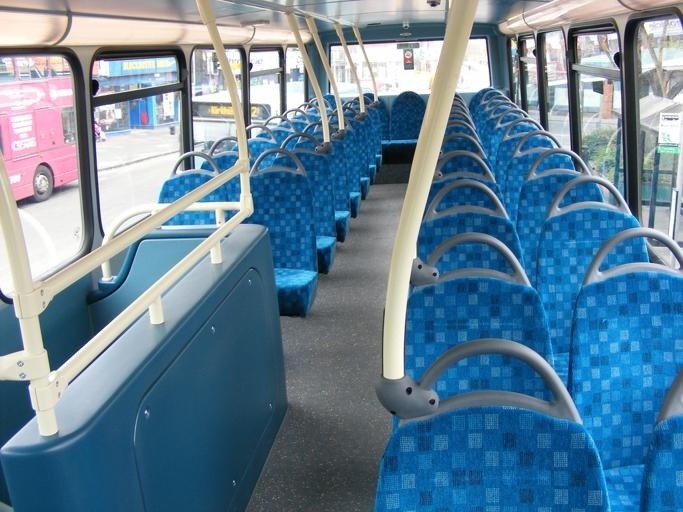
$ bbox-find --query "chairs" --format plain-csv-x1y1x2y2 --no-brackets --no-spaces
377,90,682,511
157,92,383,318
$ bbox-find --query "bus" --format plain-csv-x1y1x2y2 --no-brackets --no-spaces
169,81,305,168
0,77,79,203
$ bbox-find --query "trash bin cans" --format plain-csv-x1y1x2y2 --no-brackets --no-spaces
600,78,615,120
